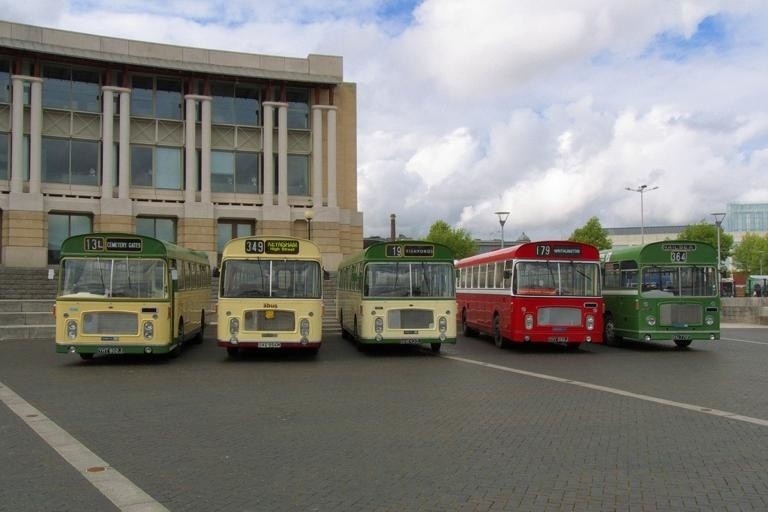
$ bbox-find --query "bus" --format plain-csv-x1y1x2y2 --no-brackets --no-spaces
452,239,606,352
596,239,720,347
47,232,212,358
212,234,330,356
334,238,460,352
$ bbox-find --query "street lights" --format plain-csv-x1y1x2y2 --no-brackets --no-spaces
494,211,512,247
304,210,314,239
710,212,726,276
625,184,659,242
